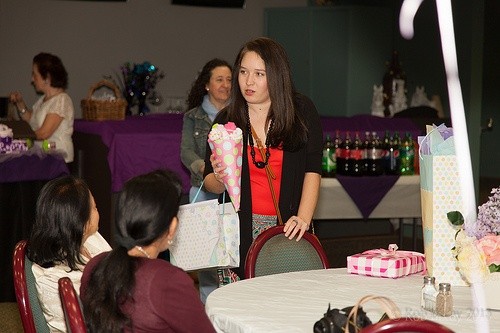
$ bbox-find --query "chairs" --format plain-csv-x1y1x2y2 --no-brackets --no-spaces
13,241,48,333
59,277,87,333
245,226,328,282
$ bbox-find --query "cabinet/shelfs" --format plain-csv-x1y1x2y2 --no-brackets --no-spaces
266,4,394,118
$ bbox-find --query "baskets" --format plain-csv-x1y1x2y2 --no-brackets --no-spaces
80,80,127,121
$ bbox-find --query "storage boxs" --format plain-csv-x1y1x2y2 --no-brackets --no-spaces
347,248,425,278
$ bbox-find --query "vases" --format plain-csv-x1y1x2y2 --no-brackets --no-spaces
483,272,500,311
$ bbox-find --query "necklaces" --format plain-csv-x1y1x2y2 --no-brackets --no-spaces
244,101,277,168
135,246,151,259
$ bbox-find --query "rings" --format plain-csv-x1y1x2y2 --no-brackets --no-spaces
293,219,298,226
213,170,217,175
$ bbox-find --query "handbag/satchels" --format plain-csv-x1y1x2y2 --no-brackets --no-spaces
313,303,373,333
418,123,472,287
168,173,241,272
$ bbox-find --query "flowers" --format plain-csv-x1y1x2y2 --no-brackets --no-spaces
465,188,500,273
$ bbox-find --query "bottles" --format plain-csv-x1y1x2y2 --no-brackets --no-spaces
436,283,452,316
321,131,416,178
421,276,437,311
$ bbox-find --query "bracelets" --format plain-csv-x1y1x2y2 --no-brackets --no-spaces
18,106,28,114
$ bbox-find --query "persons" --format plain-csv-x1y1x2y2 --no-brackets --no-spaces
9,52,75,172
80,172,217,333
179,37,323,281
23,171,100,333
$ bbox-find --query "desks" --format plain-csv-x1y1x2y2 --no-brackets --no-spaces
204,268,500,333
75,115,428,250
0,150,69,239
